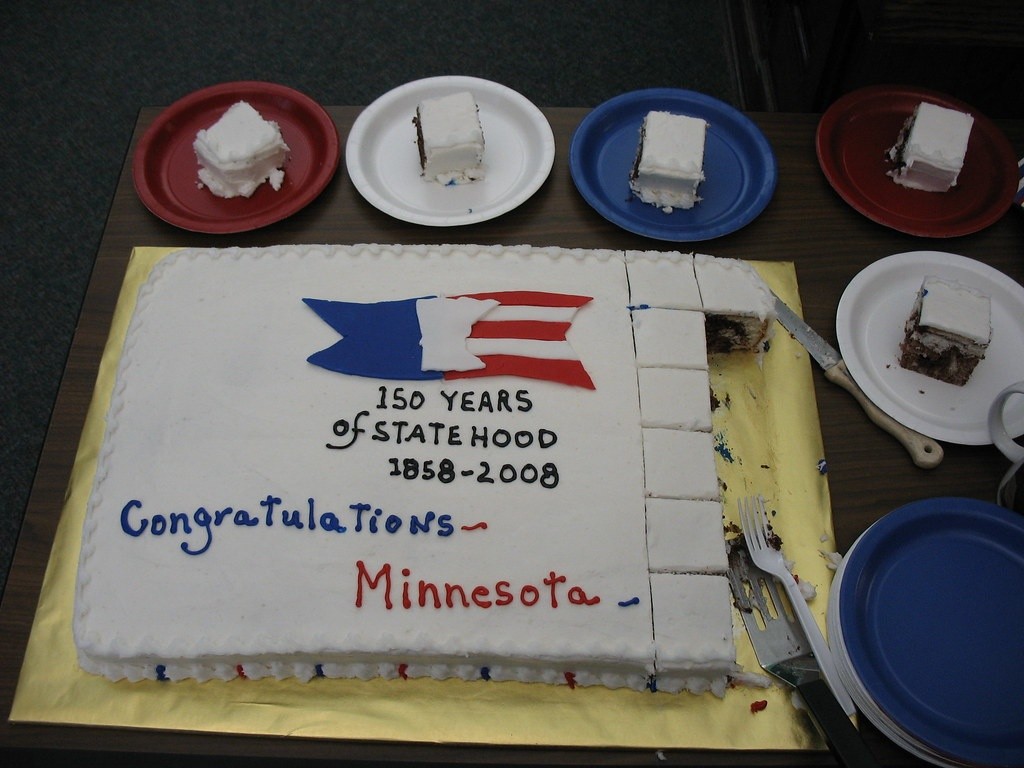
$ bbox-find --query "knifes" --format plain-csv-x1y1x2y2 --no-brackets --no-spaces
771,288,944,469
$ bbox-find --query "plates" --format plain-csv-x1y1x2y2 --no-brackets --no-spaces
836,251,1024,445
346,75,554,227
570,88,777,241
815,84,1019,237
826,496,1024,768
132,81,339,233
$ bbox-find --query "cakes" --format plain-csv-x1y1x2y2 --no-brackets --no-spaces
888,100,975,193
899,275,994,387
630,109,710,214
71,243,778,696
193,97,293,201
413,90,490,184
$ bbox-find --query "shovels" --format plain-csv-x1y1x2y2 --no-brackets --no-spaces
726,537,885,768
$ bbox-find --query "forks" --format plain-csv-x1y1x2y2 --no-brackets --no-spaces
736,496,856,717
727,564,880,768
